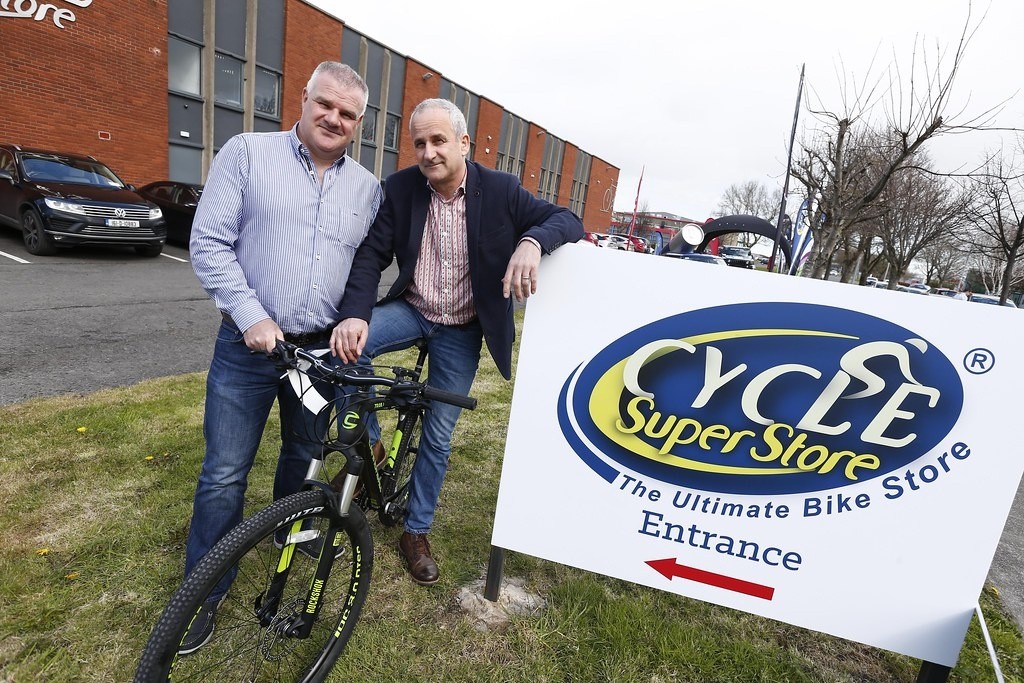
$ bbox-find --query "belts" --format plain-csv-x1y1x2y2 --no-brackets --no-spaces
220,310,333,347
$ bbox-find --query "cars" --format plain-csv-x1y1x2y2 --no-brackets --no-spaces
586,232,651,250
865,276,1018,308
0,144,168,257
721,246,756,267
131,181,205,241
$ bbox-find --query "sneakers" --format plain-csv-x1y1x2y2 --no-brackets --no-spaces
177,589,232,654
273,527,345,561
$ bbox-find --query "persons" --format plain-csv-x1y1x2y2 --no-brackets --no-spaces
954,288,972,302
328,98,585,587
173,60,387,656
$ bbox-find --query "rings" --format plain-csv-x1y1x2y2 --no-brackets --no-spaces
522,277,530,279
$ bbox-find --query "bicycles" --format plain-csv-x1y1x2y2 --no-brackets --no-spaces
134,338,476,683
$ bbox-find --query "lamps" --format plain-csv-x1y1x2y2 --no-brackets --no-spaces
422,72,433,81
535,131,544,136
606,166,611,171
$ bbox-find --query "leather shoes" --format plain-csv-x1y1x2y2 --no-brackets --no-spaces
398,530,440,585
328,438,386,500
